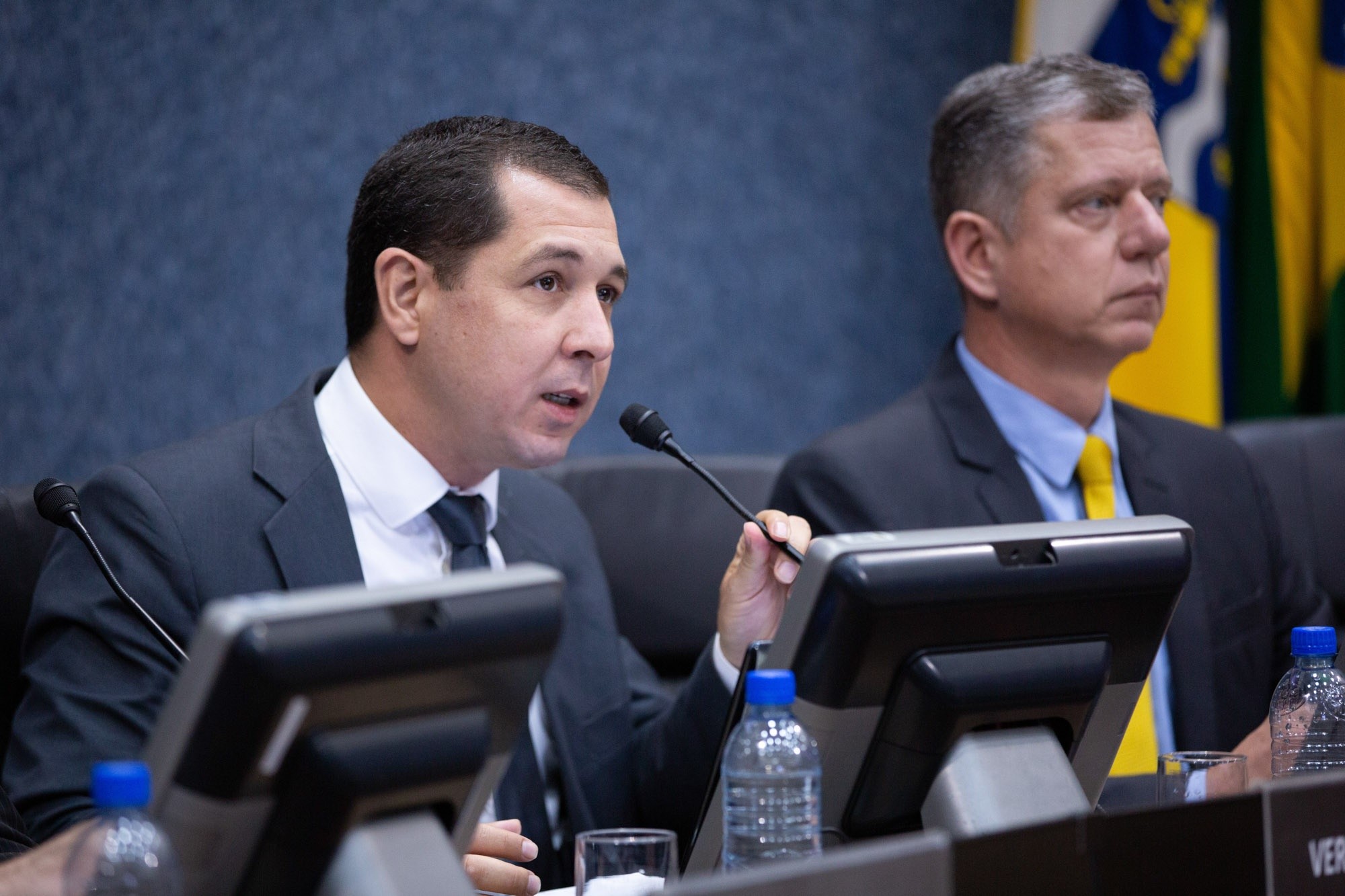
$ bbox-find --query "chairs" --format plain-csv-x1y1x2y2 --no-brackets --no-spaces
559,458,791,701
1227,412,1345,690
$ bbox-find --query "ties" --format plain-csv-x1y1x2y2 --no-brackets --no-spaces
425,489,571,892
1076,435,1159,777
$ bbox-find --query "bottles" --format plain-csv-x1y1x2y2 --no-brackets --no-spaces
719,670,822,874
62,760,186,896
1268,625,1345,781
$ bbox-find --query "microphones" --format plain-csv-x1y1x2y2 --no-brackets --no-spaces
620,403,808,565
31,478,189,665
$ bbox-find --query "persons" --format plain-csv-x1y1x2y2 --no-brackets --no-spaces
766,49,1345,813
0,114,810,896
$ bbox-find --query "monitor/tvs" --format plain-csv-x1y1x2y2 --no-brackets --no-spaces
681,515,1193,880
135,560,566,896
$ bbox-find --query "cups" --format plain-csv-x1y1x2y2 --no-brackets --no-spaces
1157,750,1248,807
573,829,680,895
